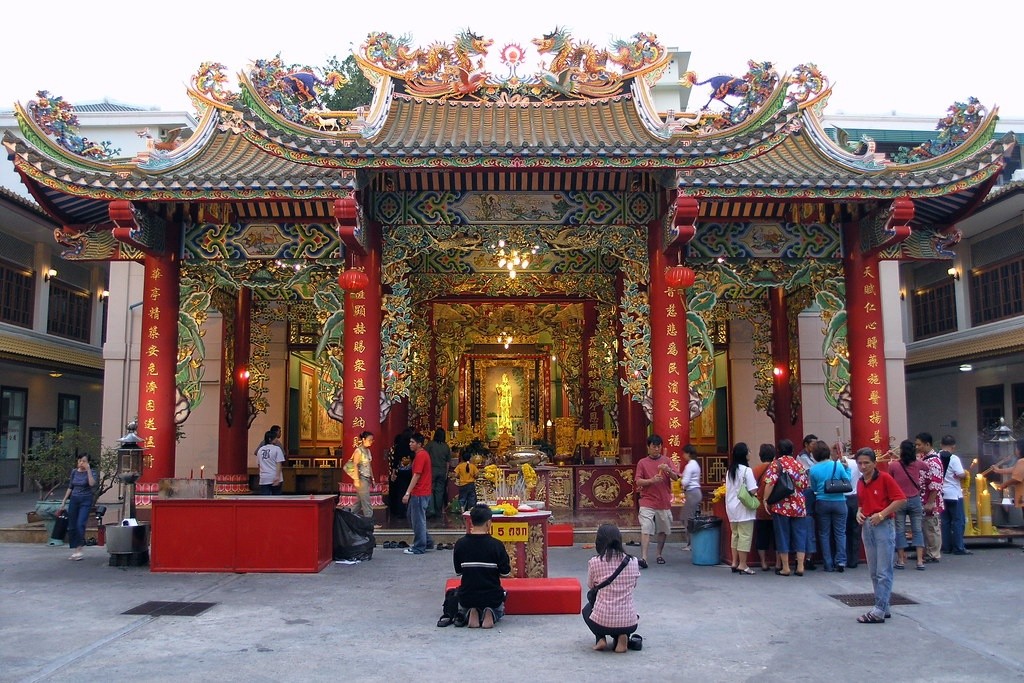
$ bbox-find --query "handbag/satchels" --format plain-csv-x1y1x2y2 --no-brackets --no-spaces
767,459,795,504
51,512,68,540
825,460,852,493
738,467,760,511
587,587,598,606
343,448,362,479
442,585,468,617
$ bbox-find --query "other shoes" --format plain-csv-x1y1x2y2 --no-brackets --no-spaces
638,557,647,568
382,540,409,549
445,543,453,550
922,554,939,564
955,549,973,556
69,554,85,560
656,557,666,564
731,557,857,577
908,552,917,559
437,542,444,550
404,549,414,554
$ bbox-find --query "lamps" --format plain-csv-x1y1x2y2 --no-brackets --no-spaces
44,270,57,282
947,268,959,281
99,291,109,302
490,231,540,278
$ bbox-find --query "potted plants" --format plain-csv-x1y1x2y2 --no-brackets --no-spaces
21,424,119,547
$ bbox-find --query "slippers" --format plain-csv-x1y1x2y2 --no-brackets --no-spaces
916,563,926,569
893,561,906,570
625,540,640,546
455,610,467,626
436,612,454,627
856,613,885,624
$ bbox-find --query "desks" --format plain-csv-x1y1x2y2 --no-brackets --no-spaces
446,465,635,579
282,467,344,494
150,495,339,573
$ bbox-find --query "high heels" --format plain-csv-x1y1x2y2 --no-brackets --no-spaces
630,633,642,650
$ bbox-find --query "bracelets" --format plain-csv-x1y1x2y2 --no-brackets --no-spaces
666,468,672,477
878,513,885,521
762,498,768,502
371,478,375,481
354,478,359,482
405,492,410,497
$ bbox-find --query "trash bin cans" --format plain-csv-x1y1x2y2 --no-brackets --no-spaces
689,515,721,566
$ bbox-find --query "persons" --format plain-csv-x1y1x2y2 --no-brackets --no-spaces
61,453,98,560
724,434,968,576
254,425,286,495
350,431,382,529
634,434,702,569
454,504,511,628
388,427,451,518
582,523,641,653
402,433,435,554
854,448,908,623
991,440,1024,553
495,373,512,436
454,451,479,513
